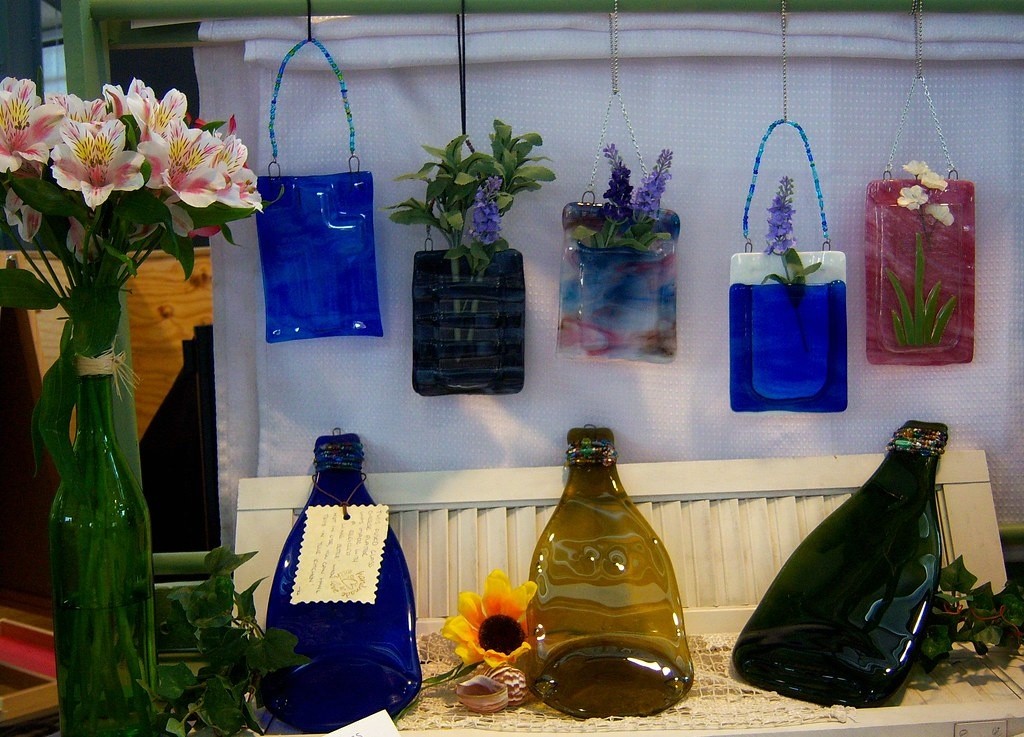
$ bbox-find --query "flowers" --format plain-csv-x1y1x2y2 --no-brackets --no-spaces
896,159,954,250
760,175,822,353
389,570,539,729
571,142,674,256
0,77,263,737
386,117,556,364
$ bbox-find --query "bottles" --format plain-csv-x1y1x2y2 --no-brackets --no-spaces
525,428,693,717
732,421,949,709
258,432,421,732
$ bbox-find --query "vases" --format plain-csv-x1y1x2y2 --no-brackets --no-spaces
48,351,154,736
